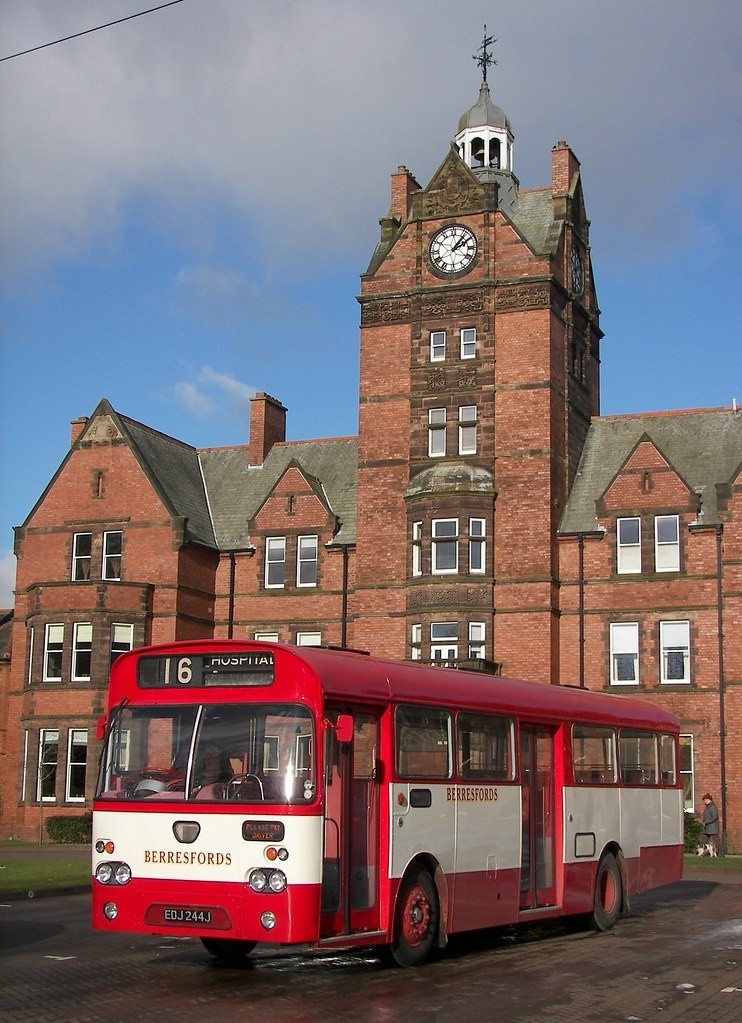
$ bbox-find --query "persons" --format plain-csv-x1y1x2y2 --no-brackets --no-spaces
694,794,722,853
168,719,233,790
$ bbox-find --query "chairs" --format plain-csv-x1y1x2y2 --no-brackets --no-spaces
462,763,673,825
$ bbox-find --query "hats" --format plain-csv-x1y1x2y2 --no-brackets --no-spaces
702,793,712,800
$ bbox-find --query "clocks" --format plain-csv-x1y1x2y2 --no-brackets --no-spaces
430,225,478,274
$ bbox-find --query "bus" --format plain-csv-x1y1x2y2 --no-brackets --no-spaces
86,637,689,964
